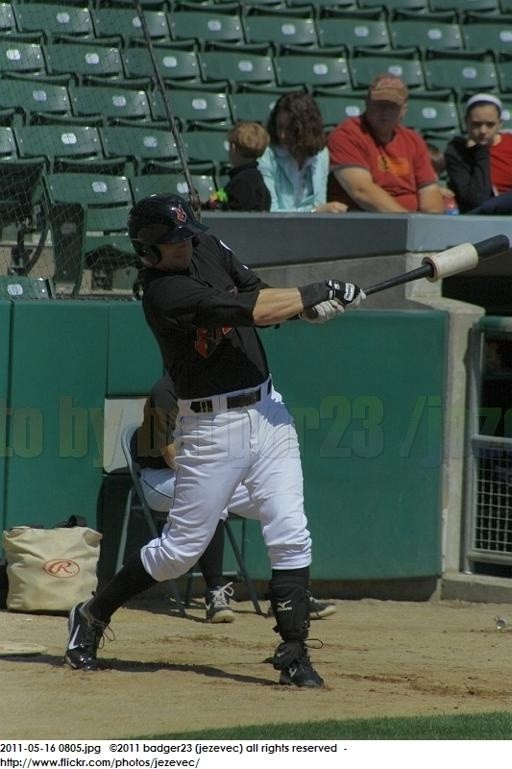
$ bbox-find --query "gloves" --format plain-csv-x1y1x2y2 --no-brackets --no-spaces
297,277,366,310
288,300,345,325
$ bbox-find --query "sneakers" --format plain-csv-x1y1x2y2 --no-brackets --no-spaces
266,598,337,620
278,656,324,688
203,578,238,623
65,598,118,671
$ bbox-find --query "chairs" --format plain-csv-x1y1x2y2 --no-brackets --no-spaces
113,422,267,619
1,1,511,286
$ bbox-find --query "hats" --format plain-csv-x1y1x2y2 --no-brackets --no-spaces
367,75,409,108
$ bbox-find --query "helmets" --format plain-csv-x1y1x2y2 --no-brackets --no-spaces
126,193,209,261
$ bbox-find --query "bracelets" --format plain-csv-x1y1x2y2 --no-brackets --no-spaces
297,280,331,309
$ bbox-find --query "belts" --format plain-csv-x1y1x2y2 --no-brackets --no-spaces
189,379,272,414
142,458,170,470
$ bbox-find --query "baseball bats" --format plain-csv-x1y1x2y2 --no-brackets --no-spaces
307,233,509,320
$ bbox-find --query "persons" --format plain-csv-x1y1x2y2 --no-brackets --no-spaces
257,92,349,214
446,94,512,216
135,372,336,623
65,192,367,690
426,143,460,216
207,121,272,212
326,77,456,215
